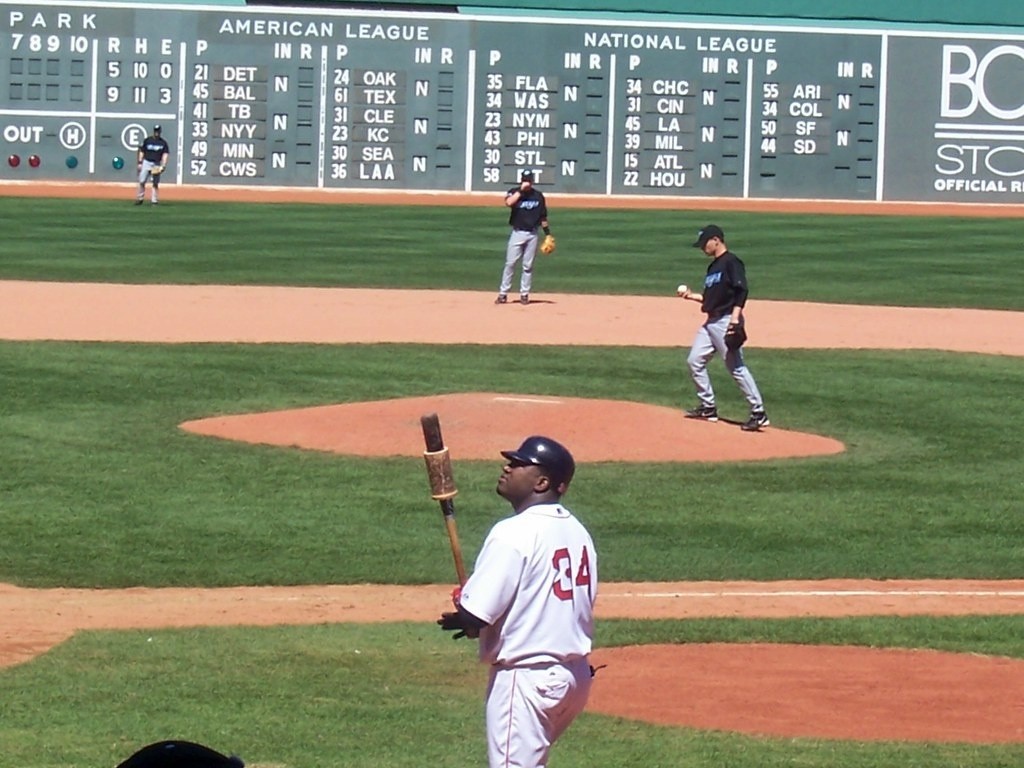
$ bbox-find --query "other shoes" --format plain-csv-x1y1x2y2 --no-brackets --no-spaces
152,202,158,206
134,200,143,205
521,294,529,305
495,294,507,304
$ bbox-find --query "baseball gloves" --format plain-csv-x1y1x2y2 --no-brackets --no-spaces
724,323,747,352
540,235,556,254
151,165,164,175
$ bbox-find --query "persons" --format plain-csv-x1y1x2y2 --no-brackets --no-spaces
495,169,555,305
437,436,600,768
678,224,770,431
134,125,169,206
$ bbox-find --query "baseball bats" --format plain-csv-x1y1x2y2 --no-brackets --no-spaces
419,412,480,639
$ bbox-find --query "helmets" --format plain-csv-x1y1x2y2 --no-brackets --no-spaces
500,435,576,494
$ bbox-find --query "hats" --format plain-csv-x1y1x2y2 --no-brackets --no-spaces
153,125,161,130
521,169,533,180
692,225,724,247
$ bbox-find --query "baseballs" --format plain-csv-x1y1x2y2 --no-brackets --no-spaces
677,285,686,292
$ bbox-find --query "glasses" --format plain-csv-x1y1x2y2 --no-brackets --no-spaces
522,179,531,182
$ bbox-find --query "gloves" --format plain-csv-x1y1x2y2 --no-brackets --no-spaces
725,323,746,352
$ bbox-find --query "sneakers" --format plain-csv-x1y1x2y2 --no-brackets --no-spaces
686,404,719,422
741,411,770,431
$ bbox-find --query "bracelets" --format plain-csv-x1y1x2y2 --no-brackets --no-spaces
138,161,142,164
518,188,522,194
